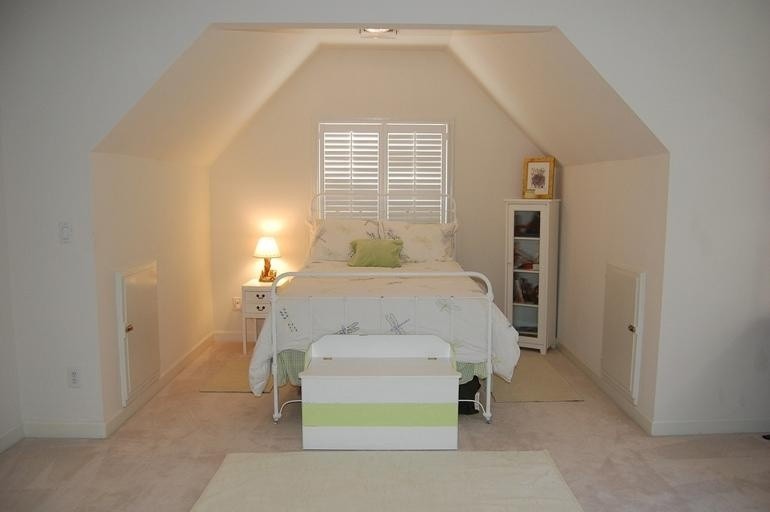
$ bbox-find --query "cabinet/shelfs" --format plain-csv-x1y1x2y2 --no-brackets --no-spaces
505,198,560,355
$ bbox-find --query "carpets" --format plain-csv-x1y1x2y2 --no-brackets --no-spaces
491,350,583,403
189,452,584,512
200,352,274,393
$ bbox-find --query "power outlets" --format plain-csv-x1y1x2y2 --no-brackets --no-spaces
233,297,240,311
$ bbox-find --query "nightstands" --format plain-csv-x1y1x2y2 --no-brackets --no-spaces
241,279,288,356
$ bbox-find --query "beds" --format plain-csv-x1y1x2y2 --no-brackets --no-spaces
272,192,494,424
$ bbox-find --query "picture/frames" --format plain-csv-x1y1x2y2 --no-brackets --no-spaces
521,156,554,199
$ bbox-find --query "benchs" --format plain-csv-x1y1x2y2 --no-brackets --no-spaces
298,335,462,451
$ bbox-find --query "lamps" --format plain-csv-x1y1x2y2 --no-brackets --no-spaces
253,238,279,282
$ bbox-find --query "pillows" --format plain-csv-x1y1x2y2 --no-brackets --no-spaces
348,238,403,267
309,219,382,261
378,219,457,263
